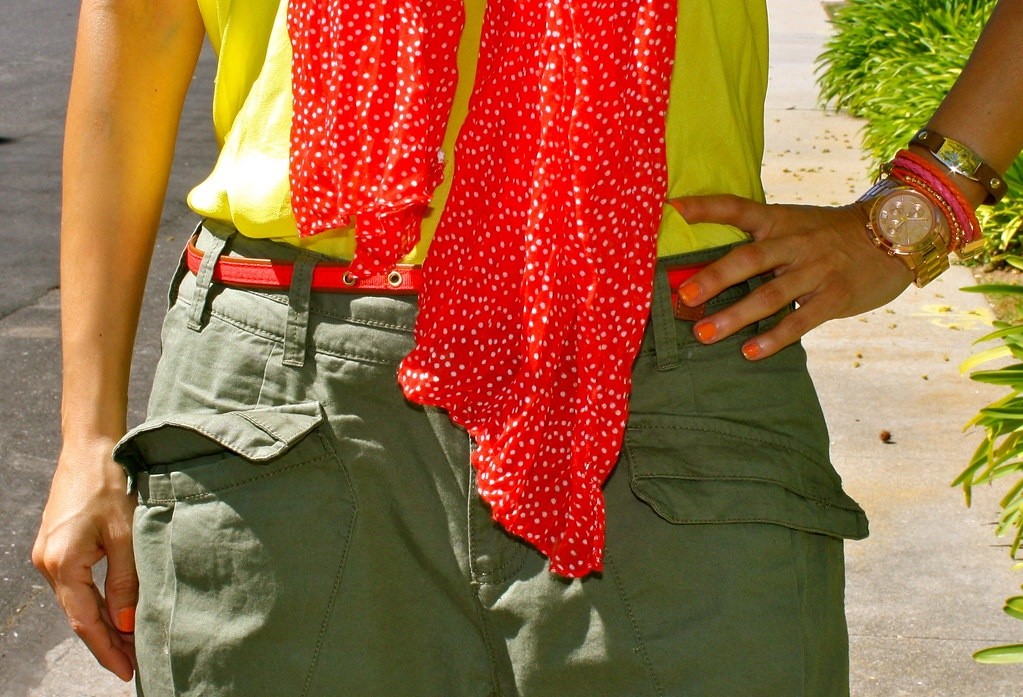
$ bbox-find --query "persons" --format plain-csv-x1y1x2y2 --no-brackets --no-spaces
31,0,1023,697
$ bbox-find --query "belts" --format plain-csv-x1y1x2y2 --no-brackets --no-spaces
188,232,736,322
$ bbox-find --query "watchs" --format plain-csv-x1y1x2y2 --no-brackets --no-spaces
855,177,952,288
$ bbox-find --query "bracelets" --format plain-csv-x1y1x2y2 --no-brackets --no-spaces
908,128,1009,206
874,147,983,253
